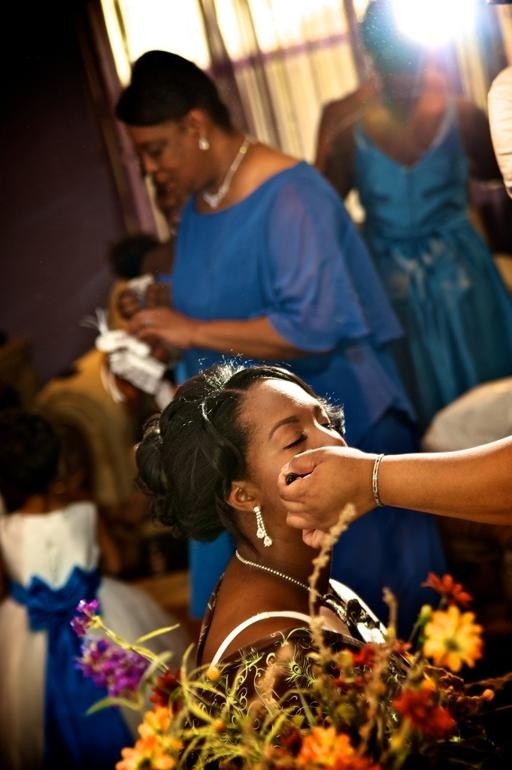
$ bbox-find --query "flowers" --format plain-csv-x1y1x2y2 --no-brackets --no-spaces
67,572,501,770
